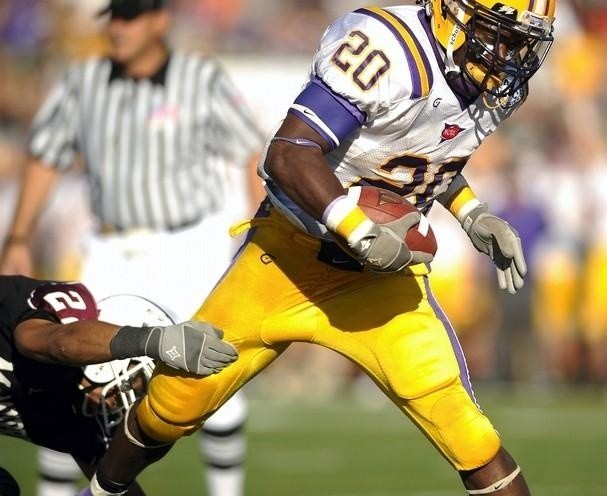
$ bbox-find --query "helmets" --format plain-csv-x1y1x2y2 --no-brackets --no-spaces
424,0,556,98
83,293,179,436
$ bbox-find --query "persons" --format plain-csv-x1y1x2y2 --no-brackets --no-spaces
97,1,566,496
0,0,272,496
0,272,242,496
0,1,606,400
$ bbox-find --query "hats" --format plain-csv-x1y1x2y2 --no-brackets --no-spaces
96,1,165,19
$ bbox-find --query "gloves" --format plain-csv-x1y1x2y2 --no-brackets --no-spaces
138,324,239,375
461,202,527,294
353,210,436,275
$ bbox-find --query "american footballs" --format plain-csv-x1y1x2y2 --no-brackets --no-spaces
344,186,437,265
